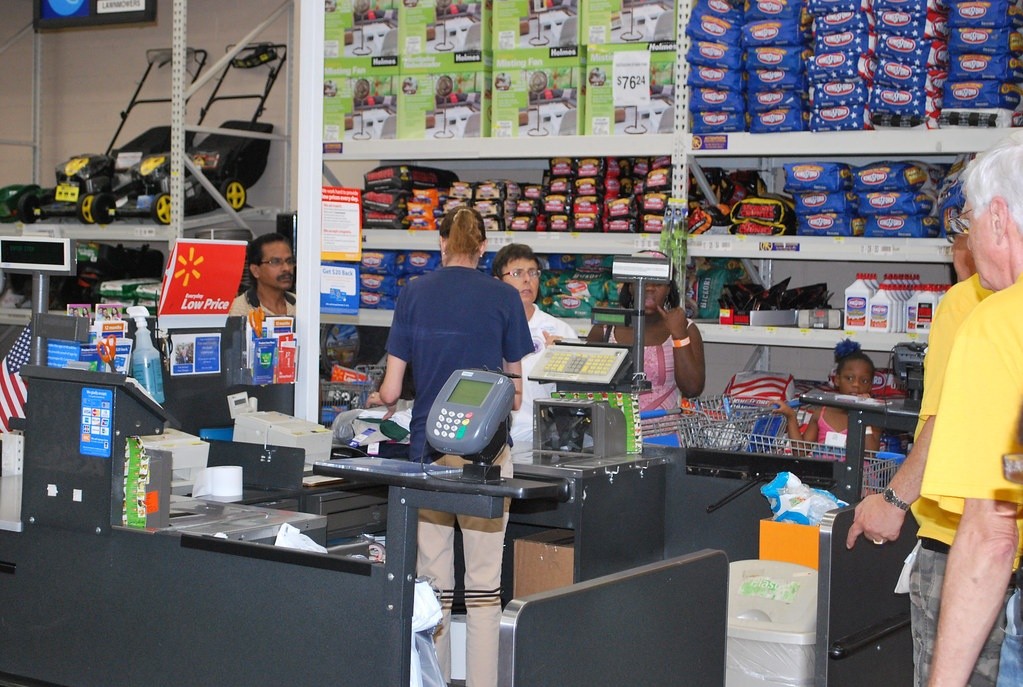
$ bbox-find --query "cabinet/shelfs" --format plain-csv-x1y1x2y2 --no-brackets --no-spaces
685,0,1023,354
319,0,683,421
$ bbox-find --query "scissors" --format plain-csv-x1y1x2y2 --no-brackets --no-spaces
97,335,117,373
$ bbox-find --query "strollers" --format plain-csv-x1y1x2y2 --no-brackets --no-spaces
17,42,287,225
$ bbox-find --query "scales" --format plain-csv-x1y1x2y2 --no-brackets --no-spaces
528,255,673,393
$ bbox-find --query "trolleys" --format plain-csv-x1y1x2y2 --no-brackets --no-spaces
319,365,385,430
639,393,906,500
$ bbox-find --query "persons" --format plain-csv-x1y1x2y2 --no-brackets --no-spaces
98,308,120,321
364,207,535,687
70,308,90,321
585,251,705,447
494,244,579,442
919,146,1023,687
229,233,297,317
847,152,1023,687
768,340,882,497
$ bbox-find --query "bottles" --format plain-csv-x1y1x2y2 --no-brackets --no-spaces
843,273,954,334
131,322,165,404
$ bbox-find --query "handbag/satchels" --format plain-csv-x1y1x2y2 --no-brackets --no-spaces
332,398,407,446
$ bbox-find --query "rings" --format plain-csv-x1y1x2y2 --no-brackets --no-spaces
873,539,883,545
371,394,374,397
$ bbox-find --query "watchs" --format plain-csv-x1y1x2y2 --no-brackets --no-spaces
883,486,910,511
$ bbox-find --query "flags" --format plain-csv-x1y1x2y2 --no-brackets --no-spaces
0,322,33,433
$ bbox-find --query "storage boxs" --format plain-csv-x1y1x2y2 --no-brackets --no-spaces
759,514,822,570
511,529,580,601
316,0,1023,431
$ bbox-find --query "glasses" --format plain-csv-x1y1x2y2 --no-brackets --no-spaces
261,258,294,267
500,269,543,279
949,208,977,235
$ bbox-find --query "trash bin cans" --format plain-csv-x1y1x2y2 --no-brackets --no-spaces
726,560,819,687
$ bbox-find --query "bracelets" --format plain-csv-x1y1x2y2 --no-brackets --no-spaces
672,336,690,348
